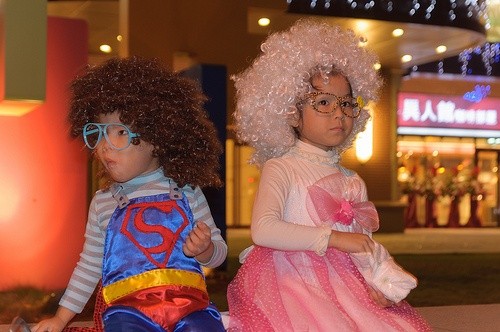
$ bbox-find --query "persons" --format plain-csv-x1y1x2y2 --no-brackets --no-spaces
30,57,227,332
227,27,434,332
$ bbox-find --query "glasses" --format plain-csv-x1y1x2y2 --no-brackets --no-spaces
294,92,363,118
83,123,141,151
